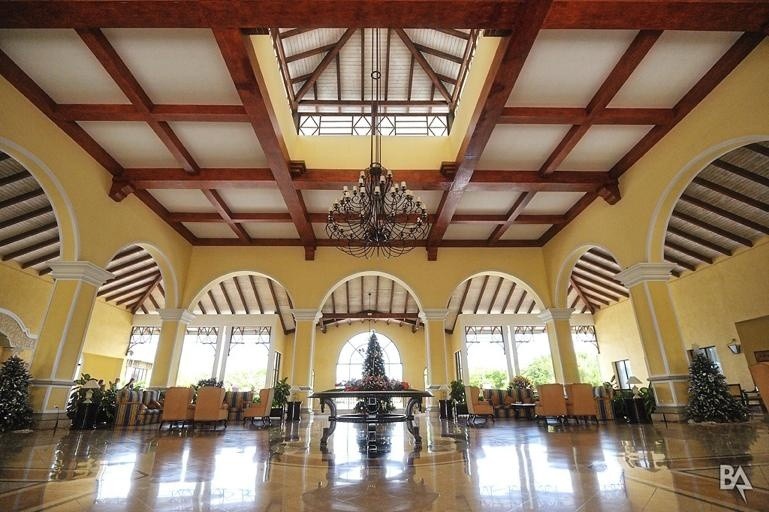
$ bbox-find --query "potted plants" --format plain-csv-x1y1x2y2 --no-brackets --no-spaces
270,377,290,420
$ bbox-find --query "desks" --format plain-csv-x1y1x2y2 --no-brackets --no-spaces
318,419,423,485
307,387,434,450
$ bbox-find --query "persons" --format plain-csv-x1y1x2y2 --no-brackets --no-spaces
125,378,136,391
98,379,108,391
109,377,122,391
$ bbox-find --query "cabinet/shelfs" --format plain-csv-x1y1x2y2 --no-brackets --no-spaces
286,400,303,421
611,398,655,423
74,401,102,429
439,400,454,420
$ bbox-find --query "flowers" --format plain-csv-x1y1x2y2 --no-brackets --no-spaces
506,375,530,393
335,376,410,390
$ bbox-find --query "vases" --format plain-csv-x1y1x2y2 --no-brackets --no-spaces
517,390,525,403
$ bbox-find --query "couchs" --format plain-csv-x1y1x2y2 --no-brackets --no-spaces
243,388,274,426
533,383,568,425
463,385,496,424
189,390,254,422
112,389,164,426
189,386,230,429
483,387,536,419
157,387,194,431
568,383,600,425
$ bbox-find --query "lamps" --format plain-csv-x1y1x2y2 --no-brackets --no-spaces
288,384,302,400
81,379,100,403
727,338,741,353
326,27,432,263
627,376,644,399
437,384,451,400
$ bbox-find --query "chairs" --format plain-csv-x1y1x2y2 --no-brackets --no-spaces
745,383,764,406
748,362,769,412
727,383,744,409
